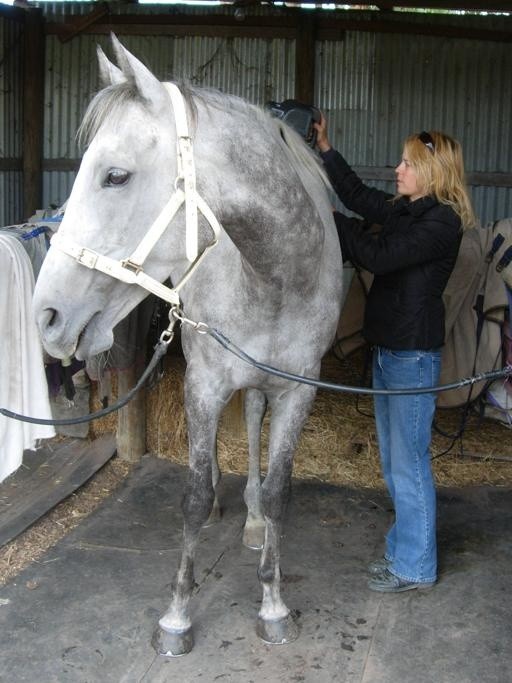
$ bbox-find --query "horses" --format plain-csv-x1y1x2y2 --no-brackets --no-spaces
31,29,355,658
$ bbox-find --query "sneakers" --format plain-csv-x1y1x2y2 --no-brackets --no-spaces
367,557,438,592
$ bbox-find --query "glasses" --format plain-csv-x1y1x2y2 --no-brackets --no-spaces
420,131,435,152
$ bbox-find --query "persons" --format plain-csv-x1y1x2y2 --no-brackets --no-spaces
310,106,478,596
